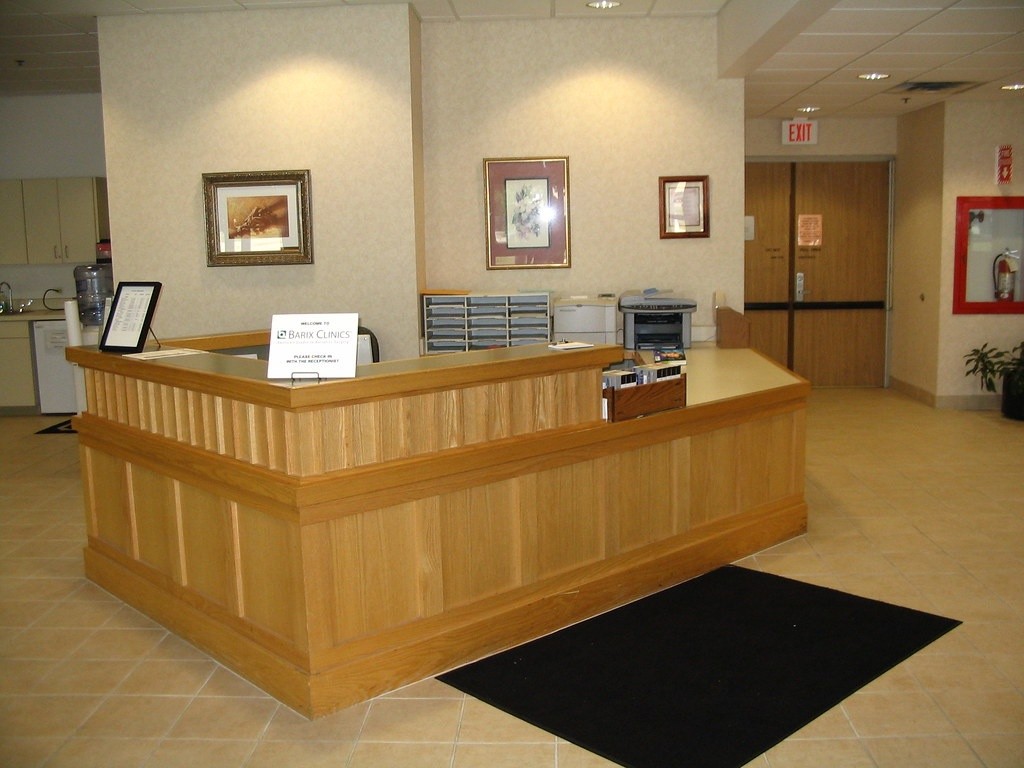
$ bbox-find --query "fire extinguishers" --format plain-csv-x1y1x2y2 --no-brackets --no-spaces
992,247,1020,302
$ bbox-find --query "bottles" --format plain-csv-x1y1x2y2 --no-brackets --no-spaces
654,346,666,363
73,264,113,326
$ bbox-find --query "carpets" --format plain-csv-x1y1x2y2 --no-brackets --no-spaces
434,561,963,768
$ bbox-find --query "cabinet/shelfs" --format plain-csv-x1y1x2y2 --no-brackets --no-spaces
0,178,29,265
423,289,553,355
553,294,625,347
22,177,110,265
0,320,41,417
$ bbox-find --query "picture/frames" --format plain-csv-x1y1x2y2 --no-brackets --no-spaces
482,156,572,271
201,169,315,268
658,174,710,239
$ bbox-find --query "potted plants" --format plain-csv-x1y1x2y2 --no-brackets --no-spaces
962,341,1024,422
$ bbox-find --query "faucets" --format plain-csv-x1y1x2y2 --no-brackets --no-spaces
0,281,13,313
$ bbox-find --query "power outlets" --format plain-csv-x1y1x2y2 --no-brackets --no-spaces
55,287,64,296
